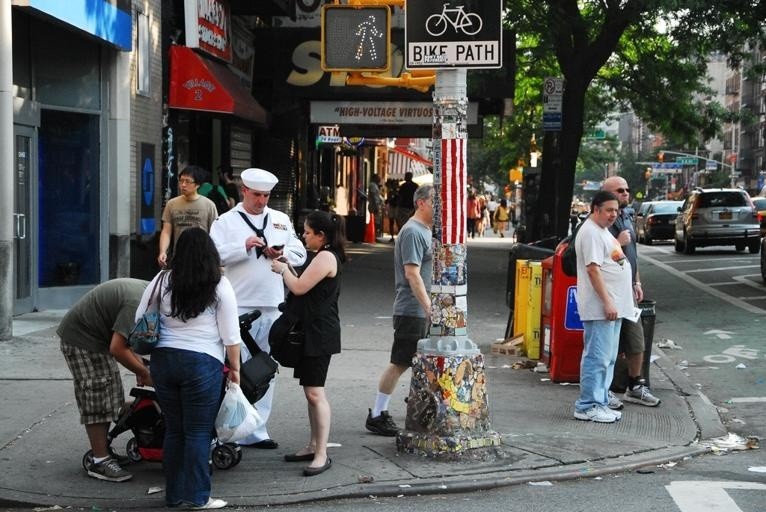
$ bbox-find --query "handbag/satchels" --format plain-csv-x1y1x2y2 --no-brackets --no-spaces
125,309,159,354
268,316,306,360
240,348,278,404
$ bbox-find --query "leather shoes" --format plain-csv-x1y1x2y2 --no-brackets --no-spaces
240,436,281,450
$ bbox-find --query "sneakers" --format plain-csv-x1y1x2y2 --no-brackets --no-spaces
364,407,405,437
573,389,624,424
191,495,230,510
111,448,132,465
85,460,132,483
621,383,663,408
165,495,190,506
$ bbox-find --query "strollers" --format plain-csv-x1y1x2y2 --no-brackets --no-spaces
80,308,280,479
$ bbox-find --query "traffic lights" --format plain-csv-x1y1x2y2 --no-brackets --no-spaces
319,4,391,73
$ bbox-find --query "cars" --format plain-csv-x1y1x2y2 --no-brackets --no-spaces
633,187,766,285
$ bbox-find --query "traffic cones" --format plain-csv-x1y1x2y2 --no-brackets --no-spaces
364,212,377,245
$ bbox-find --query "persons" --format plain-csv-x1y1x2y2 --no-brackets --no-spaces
364,184,434,438
216,165,240,209
55,277,154,483
572,190,635,425
197,168,231,216
601,175,662,411
270,209,347,477
134,226,243,510
365,169,591,245
209,167,307,450
157,165,218,270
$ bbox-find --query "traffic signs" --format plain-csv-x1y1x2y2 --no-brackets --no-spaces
581,125,606,139
650,161,683,173
677,156,699,164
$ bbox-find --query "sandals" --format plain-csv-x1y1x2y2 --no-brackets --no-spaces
284,446,321,459
301,455,333,475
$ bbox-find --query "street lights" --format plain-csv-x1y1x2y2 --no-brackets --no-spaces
648,134,669,201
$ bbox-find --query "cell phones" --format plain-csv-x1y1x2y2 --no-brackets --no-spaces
271,245,283,251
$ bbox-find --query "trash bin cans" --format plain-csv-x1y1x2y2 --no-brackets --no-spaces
343,215,365,243
609,299,656,393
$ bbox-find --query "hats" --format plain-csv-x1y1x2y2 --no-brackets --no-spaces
239,167,279,192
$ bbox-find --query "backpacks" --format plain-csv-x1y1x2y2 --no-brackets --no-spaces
562,213,625,277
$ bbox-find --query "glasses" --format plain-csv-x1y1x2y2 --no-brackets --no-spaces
178,179,196,185
609,186,631,195
